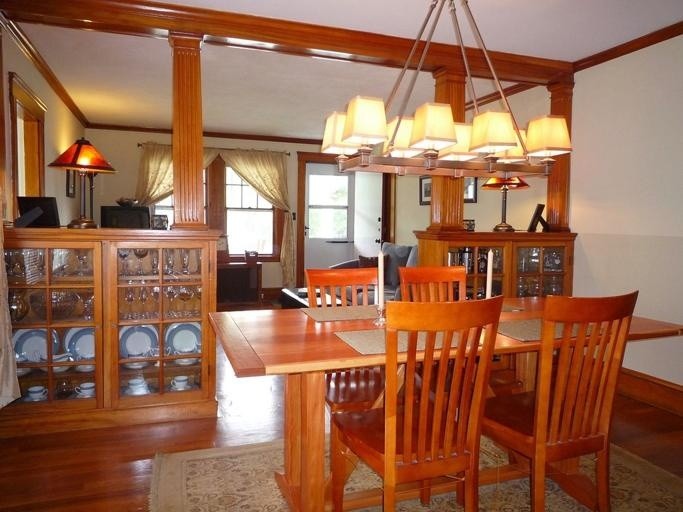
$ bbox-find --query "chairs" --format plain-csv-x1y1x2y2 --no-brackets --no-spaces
303,267,427,473
398,266,524,399
456,290,638,511
327,292,505,511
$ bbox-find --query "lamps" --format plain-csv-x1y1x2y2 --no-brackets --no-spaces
321,0,578,177
479,174,536,234
42,136,117,229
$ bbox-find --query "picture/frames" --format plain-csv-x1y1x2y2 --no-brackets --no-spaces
63,167,77,199
417,175,478,206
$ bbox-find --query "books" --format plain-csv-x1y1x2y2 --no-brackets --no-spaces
296,288,321,298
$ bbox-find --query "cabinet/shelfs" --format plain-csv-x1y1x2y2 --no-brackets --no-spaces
411,227,579,301
0,224,226,439
216,260,264,303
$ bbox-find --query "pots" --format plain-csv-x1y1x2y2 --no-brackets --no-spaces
447,252,461,266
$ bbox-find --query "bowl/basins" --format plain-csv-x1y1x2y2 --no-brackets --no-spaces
31,291,75,320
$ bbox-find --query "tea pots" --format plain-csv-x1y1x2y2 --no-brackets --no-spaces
149,344,172,367
478,258,487,272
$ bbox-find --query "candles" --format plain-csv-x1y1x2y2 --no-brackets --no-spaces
484,247,494,299
378,247,385,310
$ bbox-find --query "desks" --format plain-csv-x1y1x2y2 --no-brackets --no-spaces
207,296,682,512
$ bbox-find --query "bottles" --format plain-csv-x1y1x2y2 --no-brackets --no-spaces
459,247,473,273
9,290,30,321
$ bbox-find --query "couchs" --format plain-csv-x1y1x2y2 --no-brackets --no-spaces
324,240,416,305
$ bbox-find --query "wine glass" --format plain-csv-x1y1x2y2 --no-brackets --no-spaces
4,248,89,277
124,287,201,319
76,291,94,321
118,249,202,276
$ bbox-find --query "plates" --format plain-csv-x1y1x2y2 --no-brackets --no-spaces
176,358,196,365
122,363,148,370
171,384,192,391
124,390,150,396
62,328,96,361
164,321,203,366
12,329,59,362
118,325,158,366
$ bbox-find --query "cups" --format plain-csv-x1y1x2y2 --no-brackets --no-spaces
128,379,145,393
14,354,95,401
173,349,194,363
127,352,148,365
194,343,202,364
171,376,188,390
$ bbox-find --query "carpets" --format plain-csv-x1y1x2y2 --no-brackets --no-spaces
141,420,682,511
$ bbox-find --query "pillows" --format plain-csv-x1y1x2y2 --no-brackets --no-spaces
352,251,392,286
